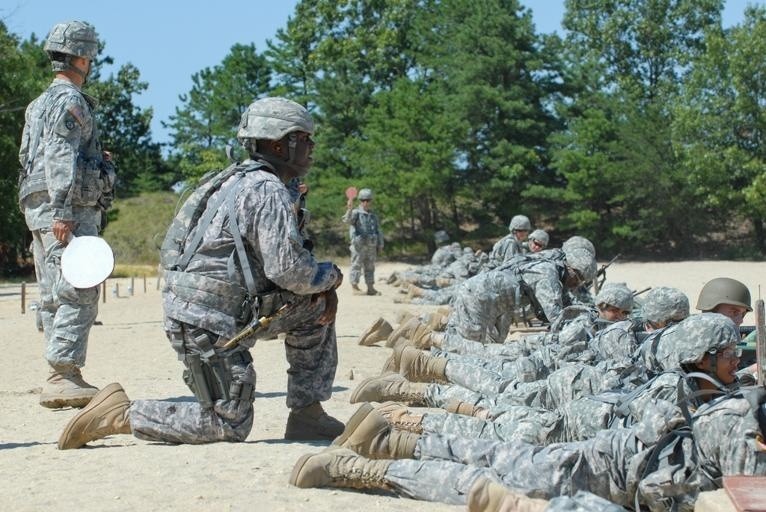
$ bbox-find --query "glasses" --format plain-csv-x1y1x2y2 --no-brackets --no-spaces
533,239,543,248
517,229,528,232
717,347,743,358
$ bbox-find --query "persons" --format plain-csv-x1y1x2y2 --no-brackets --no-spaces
16,17,118,407
342,188,384,296
289,215,766,511
57,98,345,450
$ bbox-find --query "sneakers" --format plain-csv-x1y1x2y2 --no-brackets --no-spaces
395,310,413,324
352,272,449,303
425,306,452,331
466,476,548,512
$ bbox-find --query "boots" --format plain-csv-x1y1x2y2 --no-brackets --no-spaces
358,317,392,346
368,400,428,435
55,382,132,450
329,402,417,458
350,370,428,407
284,402,346,442
378,336,448,385
444,400,490,420
387,312,442,349
287,446,394,494
39,362,99,411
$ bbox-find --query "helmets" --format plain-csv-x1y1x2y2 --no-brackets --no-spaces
358,188,373,201
508,214,531,233
234,97,315,145
562,235,596,258
598,280,633,313
529,227,548,246
669,312,740,363
43,19,99,59
641,286,689,325
433,231,481,259
565,247,596,282
694,277,753,317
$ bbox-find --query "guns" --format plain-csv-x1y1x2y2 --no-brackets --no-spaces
582,252,622,292
